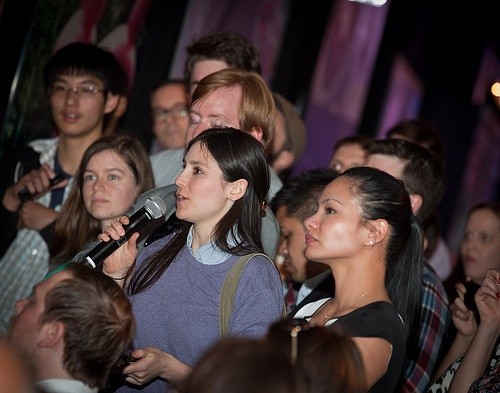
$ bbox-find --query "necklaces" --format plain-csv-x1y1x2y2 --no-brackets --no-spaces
322,287,389,321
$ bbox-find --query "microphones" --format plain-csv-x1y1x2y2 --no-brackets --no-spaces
80,195,166,269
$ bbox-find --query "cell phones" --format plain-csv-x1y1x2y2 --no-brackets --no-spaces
18,174,63,201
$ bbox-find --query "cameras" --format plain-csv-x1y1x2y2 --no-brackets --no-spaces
464,282,481,310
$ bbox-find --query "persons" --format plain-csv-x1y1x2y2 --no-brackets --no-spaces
0,31,500,393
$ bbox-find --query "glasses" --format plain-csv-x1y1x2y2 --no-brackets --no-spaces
153,105,189,123
51,80,107,97
278,318,310,367
330,160,357,174
